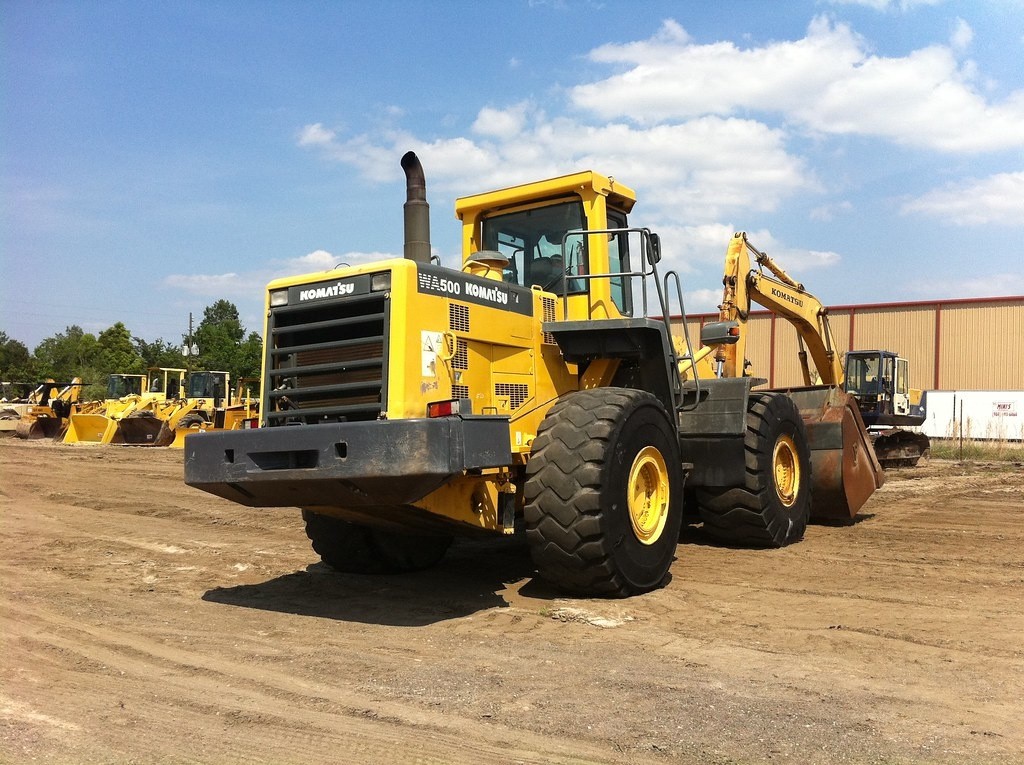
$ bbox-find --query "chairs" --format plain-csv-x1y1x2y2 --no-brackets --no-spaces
867,375,886,393
527,256,578,293
168,379,177,398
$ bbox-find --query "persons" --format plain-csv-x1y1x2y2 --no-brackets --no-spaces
551,254,581,291
167,379,178,399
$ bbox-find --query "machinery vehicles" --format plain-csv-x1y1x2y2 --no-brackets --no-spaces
0,367,262,451
711,230,932,471
183,149,889,600
